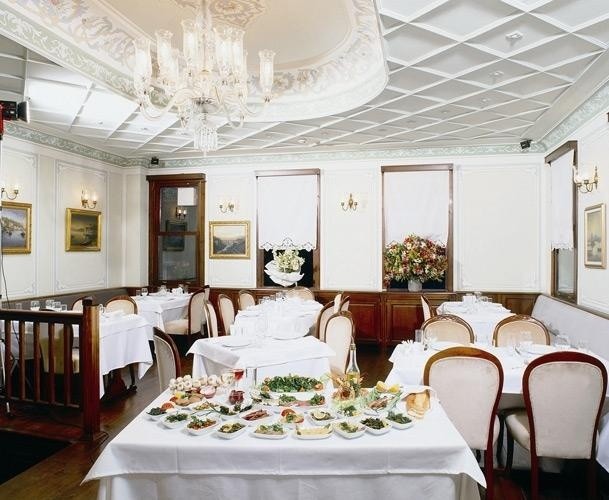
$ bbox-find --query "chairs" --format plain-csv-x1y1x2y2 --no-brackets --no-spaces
71,295,84,315
419,295,435,324
216,292,237,336
323,309,355,388
31,306,81,414
314,298,336,342
202,298,218,338
337,295,351,313
504,349,609,500
286,286,315,302
421,345,505,500
151,326,182,398
417,312,476,346
183,284,210,319
490,313,550,349
163,288,207,359
125,284,154,299
236,290,257,313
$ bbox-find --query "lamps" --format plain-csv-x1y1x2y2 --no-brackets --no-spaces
218,199,238,215
341,192,359,214
127,0,278,162
0,98,32,126
571,162,599,194
174,205,188,219
80,186,99,210
0,180,22,200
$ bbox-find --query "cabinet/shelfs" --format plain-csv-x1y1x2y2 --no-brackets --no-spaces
103,294,138,392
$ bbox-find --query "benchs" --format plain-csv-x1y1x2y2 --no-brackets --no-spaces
529,292,608,361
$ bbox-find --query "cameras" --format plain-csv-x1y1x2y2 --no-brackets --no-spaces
1,100,30,124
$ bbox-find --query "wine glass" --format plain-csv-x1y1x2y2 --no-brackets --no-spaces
220,369,234,393
233,367,244,388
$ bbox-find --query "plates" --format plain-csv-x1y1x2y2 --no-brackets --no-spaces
239,408,275,426
216,419,248,439
278,410,305,429
307,408,335,424
188,400,223,414
294,424,332,439
160,411,189,429
333,404,363,419
216,404,245,420
255,382,327,397
333,419,367,439
386,412,416,430
252,424,290,440
186,417,221,435
360,416,392,435
176,396,206,408
149,404,177,420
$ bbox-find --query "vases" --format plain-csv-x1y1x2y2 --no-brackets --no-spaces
406,275,423,293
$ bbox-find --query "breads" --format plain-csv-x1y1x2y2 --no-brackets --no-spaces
407,391,431,419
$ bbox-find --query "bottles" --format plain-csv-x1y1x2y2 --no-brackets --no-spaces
346,343,361,386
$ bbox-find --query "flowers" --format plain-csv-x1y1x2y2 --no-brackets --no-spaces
328,366,369,409
271,248,306,276
382,231,449,287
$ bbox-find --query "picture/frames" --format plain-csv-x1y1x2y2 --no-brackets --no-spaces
163,220,188,253
63,207,104,253
582,201,607,270
0,200,33,256
207,220,250,260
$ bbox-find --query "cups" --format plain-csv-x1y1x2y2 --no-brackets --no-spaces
519,329,534,346
465,290,493,311
414,329,423,343
15,299,68,312
555,334,571,350
259,290,290,309
135,284,188,298
